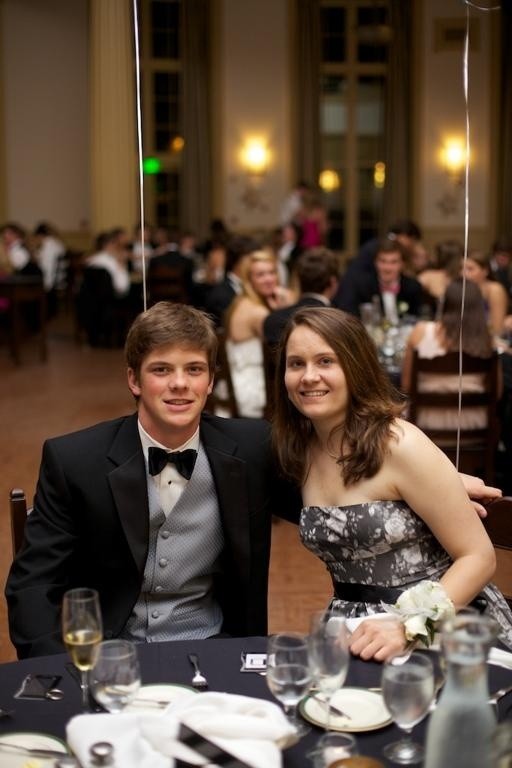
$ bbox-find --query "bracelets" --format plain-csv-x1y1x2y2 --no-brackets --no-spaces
379,579,456,650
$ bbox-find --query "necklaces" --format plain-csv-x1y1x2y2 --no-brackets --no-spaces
317,442,352,465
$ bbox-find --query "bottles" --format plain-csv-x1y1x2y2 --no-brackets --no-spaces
428,614,498,767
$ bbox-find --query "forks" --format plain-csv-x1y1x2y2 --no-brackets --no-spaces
189,653,208,690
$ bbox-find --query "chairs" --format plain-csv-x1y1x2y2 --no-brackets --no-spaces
401,348,500,487
80,269,131,347
13,279,48,359
9,487,36,560
146,252,195,308
47,253,82,312
203,333,240,420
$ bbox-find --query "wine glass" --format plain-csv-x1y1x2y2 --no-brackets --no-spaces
384,651,432,765
268,631,313,740
361,305,374,337
60,587,102,710
307,610,356,751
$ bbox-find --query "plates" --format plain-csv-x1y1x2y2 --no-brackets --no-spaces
0,733,69,768
108,685,196,712
303,686,394,731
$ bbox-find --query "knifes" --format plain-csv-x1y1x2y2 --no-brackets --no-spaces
0,742,68,759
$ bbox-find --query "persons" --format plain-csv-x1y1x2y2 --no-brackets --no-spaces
0,217,509,445
269,305,498,664
6,300,504,662
297,188,333,250
281,180,310,228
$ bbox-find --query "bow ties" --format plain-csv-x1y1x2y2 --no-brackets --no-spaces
380,284,400,294
148,447,197,480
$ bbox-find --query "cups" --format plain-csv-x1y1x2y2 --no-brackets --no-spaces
89,639,143,715
